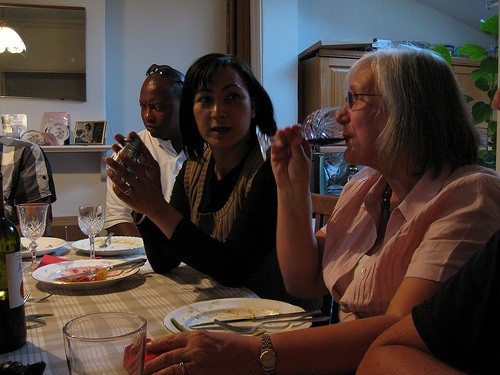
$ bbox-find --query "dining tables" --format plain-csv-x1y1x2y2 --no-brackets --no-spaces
0,241,311,375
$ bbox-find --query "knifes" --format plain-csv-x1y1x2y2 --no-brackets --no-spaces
58,258,147,280
189,308,323,328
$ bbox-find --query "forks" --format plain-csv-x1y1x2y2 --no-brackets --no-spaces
214,318,333,332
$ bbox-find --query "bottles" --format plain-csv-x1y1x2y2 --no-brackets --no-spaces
1,169,27,354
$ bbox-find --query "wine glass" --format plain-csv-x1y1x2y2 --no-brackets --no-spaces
79,203,105,259
16,204,49,273
255,106,344,160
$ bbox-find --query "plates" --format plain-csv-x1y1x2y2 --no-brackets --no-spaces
165,297,312,336
22,130,44,145
46,121,70,143
72,236,144,255
20,237,67,257
32,259,139,291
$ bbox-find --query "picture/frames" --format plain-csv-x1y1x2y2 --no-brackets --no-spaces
73,120,107,144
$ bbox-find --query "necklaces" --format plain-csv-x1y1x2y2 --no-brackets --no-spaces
382,188,395,220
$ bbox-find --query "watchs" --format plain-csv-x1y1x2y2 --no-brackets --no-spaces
258,333,276,375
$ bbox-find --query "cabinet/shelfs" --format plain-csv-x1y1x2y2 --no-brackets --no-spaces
298,49,498,154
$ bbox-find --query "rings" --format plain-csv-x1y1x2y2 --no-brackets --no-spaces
123,188,133,196
179,362,187,375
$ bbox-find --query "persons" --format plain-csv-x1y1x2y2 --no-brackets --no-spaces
80,124,93,142
0,135,57,236
357,88,500,375
140,48,500,375
104,62,195,235
105,52,313,302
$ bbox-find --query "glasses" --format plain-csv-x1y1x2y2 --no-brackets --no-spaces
146,63,184,87
345,91,381,108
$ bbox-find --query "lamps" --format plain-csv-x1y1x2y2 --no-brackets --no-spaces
0,7,26,53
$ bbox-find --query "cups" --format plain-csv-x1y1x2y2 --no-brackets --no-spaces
62,311,147,375
